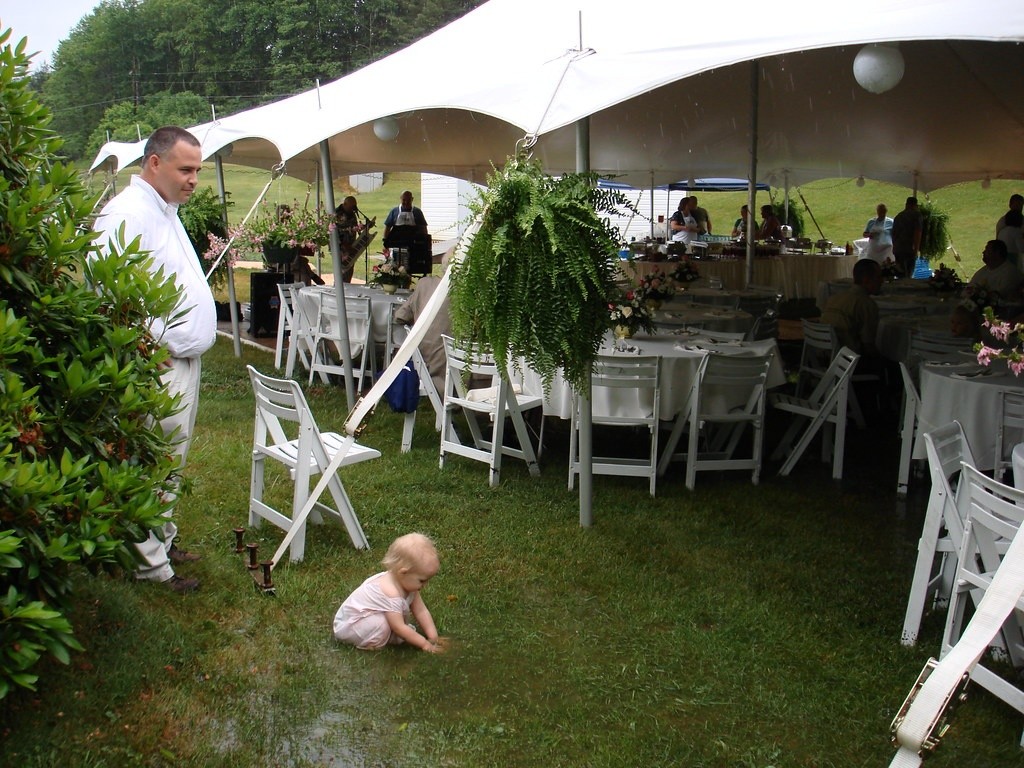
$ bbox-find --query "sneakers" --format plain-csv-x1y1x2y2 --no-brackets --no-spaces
160,575,201,595
167,537,202,565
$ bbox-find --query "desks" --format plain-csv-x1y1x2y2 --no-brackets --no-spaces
651,302,752,332
503,316,786,457
816,277,932,312
616,255,860,302
293,287,416,362
913,361,1024,473
875,312,957,364
688,290,779,305
1012,443,1024,511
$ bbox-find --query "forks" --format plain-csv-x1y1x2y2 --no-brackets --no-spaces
955,367,993,379
603,326,740,357
929,358,970,366
314,281,415,303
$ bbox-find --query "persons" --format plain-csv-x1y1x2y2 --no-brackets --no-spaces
759,205,786,247
333,533,448,653
327,196,375,283
996,194,1024,268
83,126,218,591
816,259,894,424
892,197,923,270
689,196,712,234
670,197,701,245
968,240,1024,303
863,204,895,264
394,246,509,436
731,205,759,239
383,190,429,253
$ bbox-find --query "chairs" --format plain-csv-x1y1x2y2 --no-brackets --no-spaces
244,276,1023,754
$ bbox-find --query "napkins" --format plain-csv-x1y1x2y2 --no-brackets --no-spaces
614,347,640,357
927,360,973,367
949,370,1006,380
674,343,723,355
697,337,750,347
672,326,697,336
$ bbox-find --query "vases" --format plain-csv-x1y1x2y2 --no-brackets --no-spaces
616,327,638,340
261,240,297,264
646,298,660,311
677,281,693,291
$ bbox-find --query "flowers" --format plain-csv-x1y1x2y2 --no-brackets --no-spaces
880,258,1024,377
203,190,360,270
607,254,702,336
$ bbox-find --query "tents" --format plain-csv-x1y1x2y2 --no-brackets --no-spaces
596,176,777,238
73,0,1024,768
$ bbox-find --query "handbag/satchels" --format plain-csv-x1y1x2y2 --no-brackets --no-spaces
215,301,244,321
375,360,420,414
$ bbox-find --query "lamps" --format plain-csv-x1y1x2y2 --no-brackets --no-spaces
688,178,696,188
217,144,234,157
853,42,905,94
374,117,400,140
983,179,991,187
857,176,865,187
